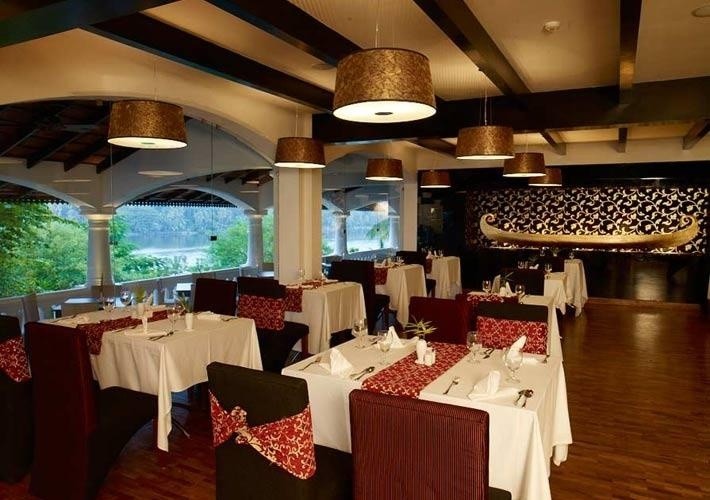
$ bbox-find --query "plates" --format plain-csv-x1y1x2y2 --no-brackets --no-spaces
283,279,338,289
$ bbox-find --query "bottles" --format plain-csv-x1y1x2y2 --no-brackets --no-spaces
416,339,436,367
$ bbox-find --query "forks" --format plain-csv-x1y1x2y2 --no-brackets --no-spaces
49,313,79,323
484,350,494,358
543,355,550,363
116,324,137,332
443,377,459,395
515,390,524,404
299,356,321,371
221,317,237,321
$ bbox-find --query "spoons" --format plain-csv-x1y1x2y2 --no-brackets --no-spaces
522,390,533,407
354,367,374,380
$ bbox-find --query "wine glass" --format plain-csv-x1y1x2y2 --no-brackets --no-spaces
342,252,404,268
353,319,369,348
377,330,393,365
502,347,524,383
518,246,575,279
467,331,482,363
120,289,133,313
483,281,526,304
298,268,305,286
104,296,115,318
426,250,444,260
167,306,179,333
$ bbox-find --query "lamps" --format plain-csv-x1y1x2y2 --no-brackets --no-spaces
455,85,516,160
107,99,188,151
527,167,565,188
364,143,404,182
332,22,439,123
274,107,327,169
420,152,452,190
502,134,547,179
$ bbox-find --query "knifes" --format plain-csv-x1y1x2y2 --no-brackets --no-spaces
148,331,173,341
484,349,491,354
370,336,378,346
349,368,369,377
